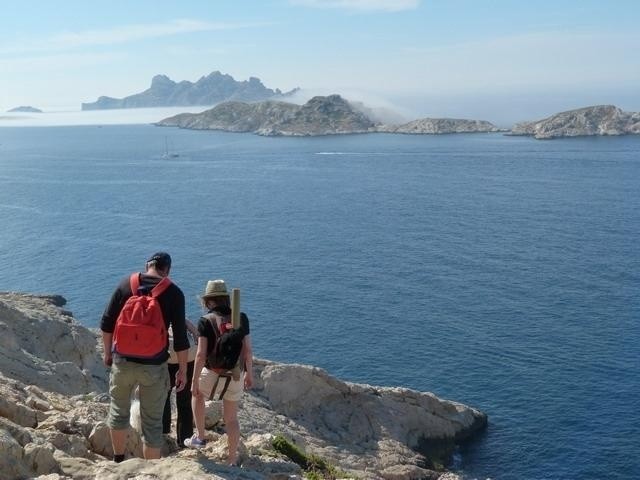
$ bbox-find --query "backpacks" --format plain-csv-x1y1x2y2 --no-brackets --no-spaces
114,272,170,357
208,325,244,375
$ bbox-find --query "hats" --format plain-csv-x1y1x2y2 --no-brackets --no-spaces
147,252,171,267
201,280,229,298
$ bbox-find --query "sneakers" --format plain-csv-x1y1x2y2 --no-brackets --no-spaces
184,434,206,448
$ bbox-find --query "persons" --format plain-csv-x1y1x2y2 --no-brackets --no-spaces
183,279,254,467
98,251,191,463
162,317,199,449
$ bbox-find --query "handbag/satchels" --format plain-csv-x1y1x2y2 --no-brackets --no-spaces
168,338,198,364
